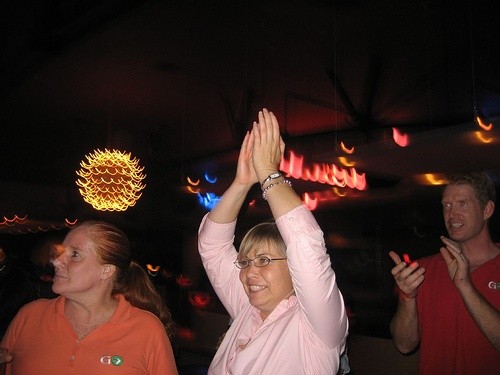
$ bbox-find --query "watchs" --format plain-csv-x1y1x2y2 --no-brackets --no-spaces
260,172,282,190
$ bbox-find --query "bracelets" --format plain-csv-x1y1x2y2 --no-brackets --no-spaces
262,179,292,200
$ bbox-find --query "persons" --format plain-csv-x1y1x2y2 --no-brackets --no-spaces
389,170,500,375
197,108,350,375
0,220,179,375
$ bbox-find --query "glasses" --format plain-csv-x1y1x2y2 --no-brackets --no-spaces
233,256,273,267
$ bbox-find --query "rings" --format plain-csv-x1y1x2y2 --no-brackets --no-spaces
459,250,463,254
